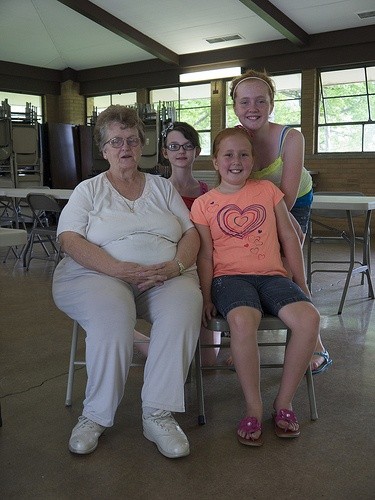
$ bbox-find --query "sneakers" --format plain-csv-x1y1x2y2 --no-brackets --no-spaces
142,408,191,459
68,415,107,455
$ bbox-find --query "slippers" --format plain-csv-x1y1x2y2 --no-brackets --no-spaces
236,417,264,447
271,407,301,438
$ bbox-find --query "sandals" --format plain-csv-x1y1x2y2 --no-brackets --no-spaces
304,352,333,376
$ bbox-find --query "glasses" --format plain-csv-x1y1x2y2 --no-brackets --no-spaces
165,142,197,151
102,135,143,148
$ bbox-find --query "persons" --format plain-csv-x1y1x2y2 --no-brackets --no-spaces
189,128,320,446
225,72,333,375
161,121,212,212
52,104,204,458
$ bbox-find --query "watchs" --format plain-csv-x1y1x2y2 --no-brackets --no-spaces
173,258,187,276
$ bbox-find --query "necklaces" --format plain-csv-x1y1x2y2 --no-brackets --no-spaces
109,170,142,212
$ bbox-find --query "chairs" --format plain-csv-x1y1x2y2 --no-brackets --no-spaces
0,98,375,424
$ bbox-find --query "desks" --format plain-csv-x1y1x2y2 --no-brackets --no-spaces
310,195,375,315
0,188,79,263
